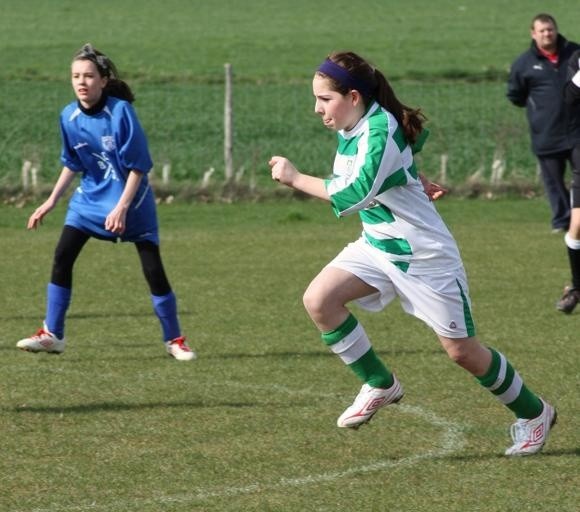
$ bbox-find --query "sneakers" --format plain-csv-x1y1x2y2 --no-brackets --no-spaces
503,396,557,456
337,372,404,431
165,336,197,361
16,321,66,354
557,287,579,313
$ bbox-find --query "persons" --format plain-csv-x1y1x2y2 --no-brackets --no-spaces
507,12,579,233
269,50,559,456
18,43,198,360
555,49,580,313
